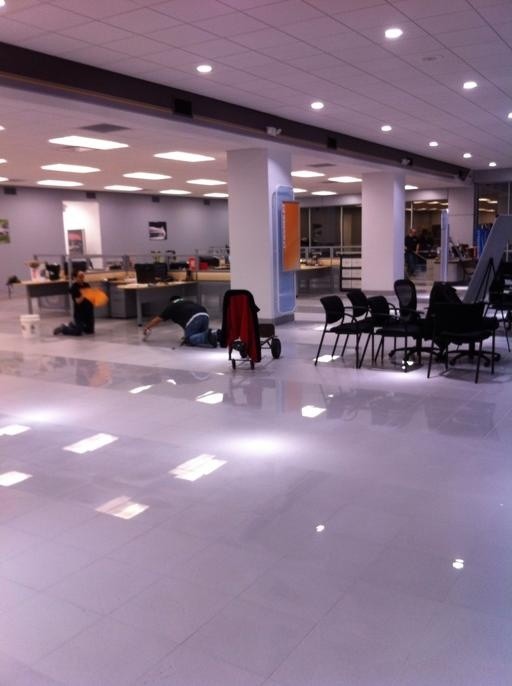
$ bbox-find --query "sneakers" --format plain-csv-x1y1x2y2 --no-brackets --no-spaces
208,329,227,349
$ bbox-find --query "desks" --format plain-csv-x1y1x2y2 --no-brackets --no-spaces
18,270,233,328
298,257,331,288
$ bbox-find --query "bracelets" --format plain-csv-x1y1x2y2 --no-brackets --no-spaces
145,326,150,329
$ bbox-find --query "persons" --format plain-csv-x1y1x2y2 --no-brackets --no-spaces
142,295,225,348
54,271,95,336
224,245,230,265
405,229,419,276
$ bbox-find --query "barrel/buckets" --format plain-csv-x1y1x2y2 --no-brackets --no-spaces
19,314,40,338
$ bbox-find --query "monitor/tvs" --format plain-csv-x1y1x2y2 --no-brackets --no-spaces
64,261,86,276
134,263,168,283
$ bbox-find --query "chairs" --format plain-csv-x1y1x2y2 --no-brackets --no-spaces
313,278,500,386
